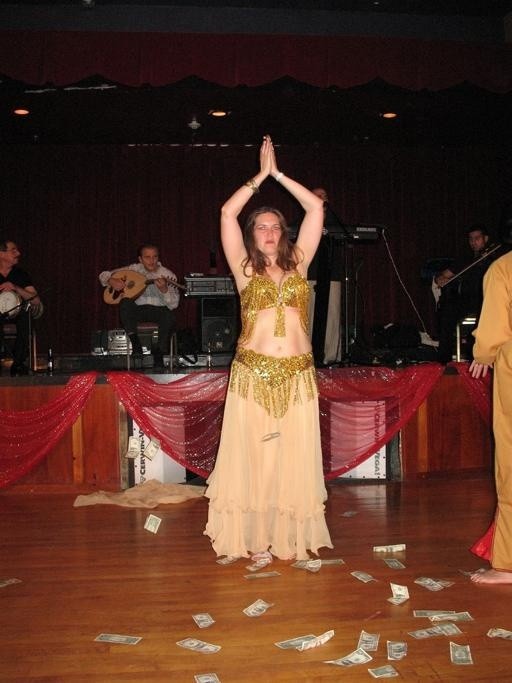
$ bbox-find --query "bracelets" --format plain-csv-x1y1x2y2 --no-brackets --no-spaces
245,177,260,192
275,172,283,181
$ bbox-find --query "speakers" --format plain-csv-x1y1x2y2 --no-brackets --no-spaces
197,296,238,353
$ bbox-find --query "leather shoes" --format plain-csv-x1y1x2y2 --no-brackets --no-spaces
8,363,50,377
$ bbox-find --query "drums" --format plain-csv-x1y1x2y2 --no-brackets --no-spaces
0,291,44,320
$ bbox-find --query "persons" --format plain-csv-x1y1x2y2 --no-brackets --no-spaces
468,250,512,584
433,226,495,360
203,134,336,562
0,239,41,363
98,243,180,360
287,186,344,354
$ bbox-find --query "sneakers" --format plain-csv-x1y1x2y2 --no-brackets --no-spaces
151,350,167,374
129,350,147,366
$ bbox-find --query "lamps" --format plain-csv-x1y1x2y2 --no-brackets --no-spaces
377,96,400,121
207,94,231,119
184,105,204,129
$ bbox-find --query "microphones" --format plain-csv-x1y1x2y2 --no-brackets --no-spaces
324,200,328,208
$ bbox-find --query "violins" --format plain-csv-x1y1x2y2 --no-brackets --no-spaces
472,242,503,266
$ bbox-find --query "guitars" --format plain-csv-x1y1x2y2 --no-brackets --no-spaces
102,270,186,304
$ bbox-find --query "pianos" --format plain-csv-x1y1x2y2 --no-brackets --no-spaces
286,225,378,239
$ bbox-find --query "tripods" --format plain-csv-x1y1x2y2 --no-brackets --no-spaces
330,213,386,368
